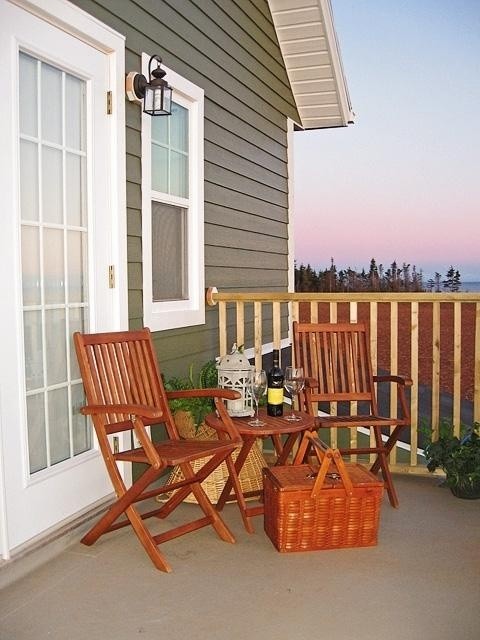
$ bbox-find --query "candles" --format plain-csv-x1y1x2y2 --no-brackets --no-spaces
226,387,245,412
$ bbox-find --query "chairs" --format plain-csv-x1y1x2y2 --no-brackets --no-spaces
73,326,243,574
292,320,413,508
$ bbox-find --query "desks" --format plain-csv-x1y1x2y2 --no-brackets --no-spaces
204,404,315,535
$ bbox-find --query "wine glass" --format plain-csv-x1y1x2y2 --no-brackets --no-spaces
283,365,306,422
245,370,269,427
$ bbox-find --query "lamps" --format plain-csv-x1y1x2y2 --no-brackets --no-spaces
125,54,173,117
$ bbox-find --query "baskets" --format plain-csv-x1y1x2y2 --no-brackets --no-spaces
155,419,271,506
261,429,386,554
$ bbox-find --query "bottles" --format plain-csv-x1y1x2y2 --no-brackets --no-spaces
267,349,285,417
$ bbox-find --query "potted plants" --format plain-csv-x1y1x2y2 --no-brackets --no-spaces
160,373,202,438
418,413,480,499
